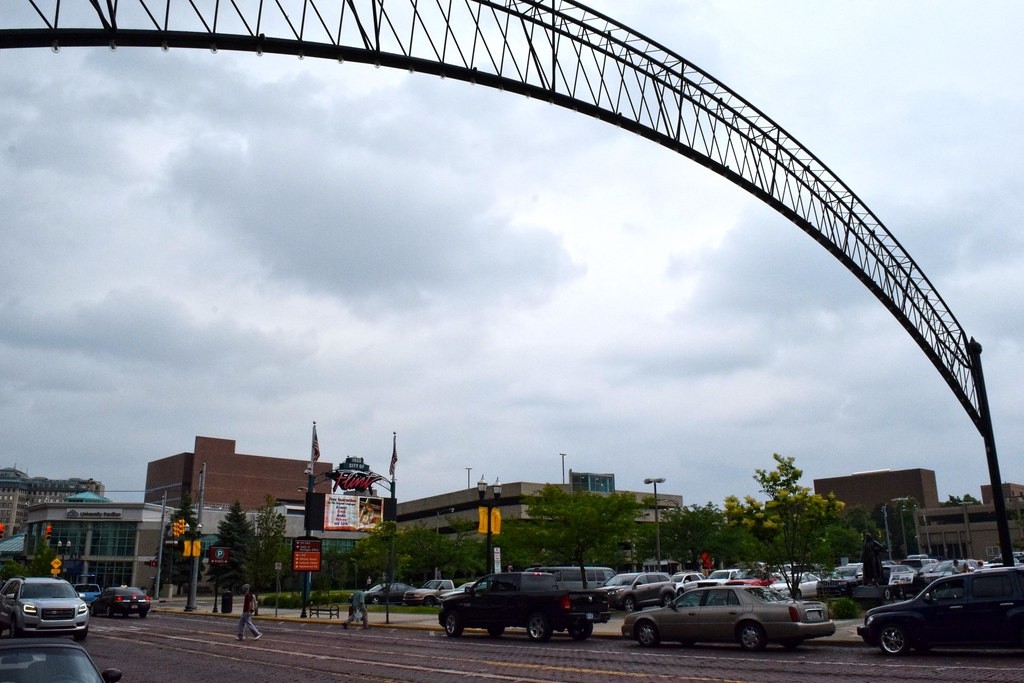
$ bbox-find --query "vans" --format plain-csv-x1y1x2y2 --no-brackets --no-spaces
526,565,619,593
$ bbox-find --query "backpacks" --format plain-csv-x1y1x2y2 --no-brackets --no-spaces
252,594,258,612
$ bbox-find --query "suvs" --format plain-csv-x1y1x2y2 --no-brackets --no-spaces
593,573,675,612
0,575,90,641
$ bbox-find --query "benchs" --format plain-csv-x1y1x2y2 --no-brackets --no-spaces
310,602,339,619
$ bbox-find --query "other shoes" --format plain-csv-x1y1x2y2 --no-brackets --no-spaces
361,625,371,630
253,633,262,641
235,637,246,640
342,622,348,629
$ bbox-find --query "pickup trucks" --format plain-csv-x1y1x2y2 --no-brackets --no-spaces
438,570,613,644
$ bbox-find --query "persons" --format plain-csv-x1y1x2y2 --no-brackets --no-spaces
951,559,960,575
235,584,262,641
978,561,984,569
341,584,372,630
860,533,885,586
366,575,371,590
961,562,973,573
508,565,513,573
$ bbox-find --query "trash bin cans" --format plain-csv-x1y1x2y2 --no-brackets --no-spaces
162,584,173,598
222,591,232,613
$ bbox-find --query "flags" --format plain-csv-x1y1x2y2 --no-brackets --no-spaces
313,426,320,464
389,435,397,475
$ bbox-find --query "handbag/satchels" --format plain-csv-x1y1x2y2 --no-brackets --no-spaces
349,606,356,616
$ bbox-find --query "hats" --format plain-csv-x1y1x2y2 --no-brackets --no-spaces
242,584,250,591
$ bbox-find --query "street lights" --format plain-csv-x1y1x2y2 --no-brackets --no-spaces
58,540,72,578
184,523,203,612
438,507,457,578
476,473,503,578
644,477,669,572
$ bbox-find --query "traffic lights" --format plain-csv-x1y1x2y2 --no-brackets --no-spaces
150,560,158,567
0,524,4,539
173,523,179,538
178,519,185,534
46,525,53,541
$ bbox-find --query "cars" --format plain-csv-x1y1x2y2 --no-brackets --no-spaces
70,583,101,609
348,581,415,605
668,550,1024,601
856,563,1024,657
89,586,151,619
403,579,459,608
618,585,838,653
437,580,484,606
0,635,123,683
